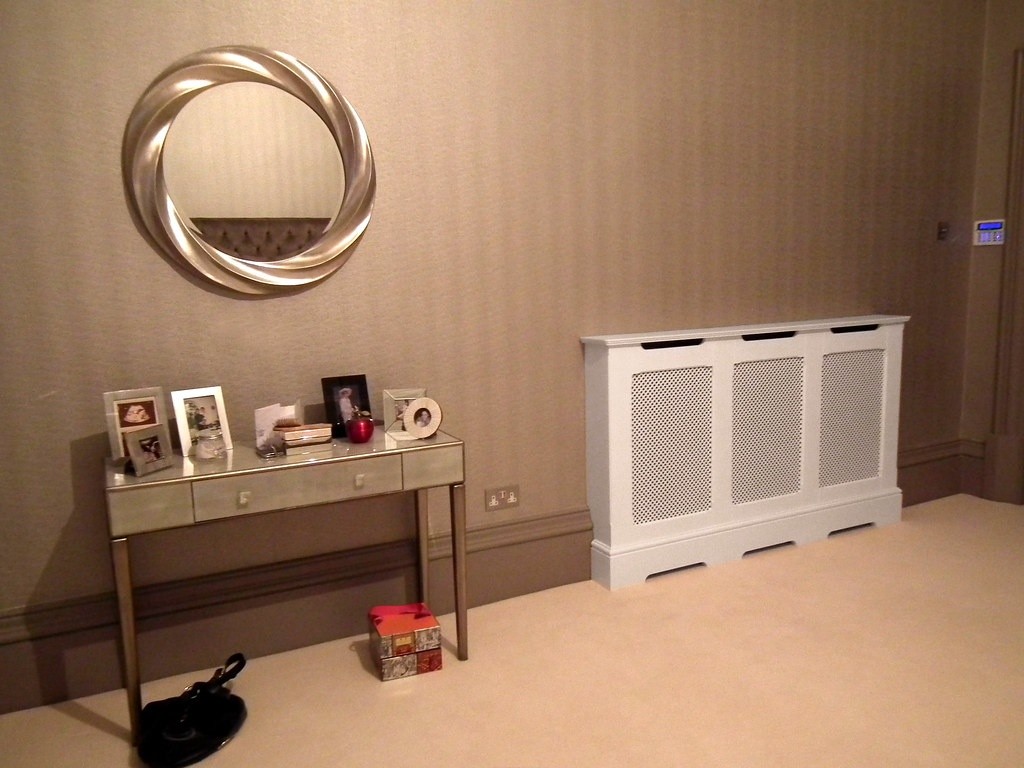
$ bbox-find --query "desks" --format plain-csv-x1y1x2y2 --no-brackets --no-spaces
104,422,469,734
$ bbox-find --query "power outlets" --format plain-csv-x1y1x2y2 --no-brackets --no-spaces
484,484,520,512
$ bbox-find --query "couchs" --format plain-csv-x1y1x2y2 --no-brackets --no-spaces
189,217,331,262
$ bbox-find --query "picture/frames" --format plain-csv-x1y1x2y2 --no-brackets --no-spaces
171,385,234,456
383,388,427,432
103,387,170,466
321,374,374,437
121,425,173,478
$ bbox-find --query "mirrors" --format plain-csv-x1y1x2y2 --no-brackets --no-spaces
123,46,376,300
